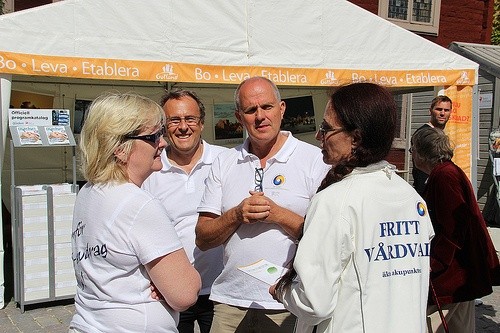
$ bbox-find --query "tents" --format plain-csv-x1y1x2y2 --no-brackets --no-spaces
0,0,480,310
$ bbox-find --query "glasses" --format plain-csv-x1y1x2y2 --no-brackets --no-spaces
408,147,418,155
124,125,166,143
167,116,202,126
255,167,263,192
319,122,347,134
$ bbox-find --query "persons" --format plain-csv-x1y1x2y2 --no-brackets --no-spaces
412,96,453,197
268,82,431,333
67,90,203,333
411,127,500,333
139,89,230,333
195,76,333,333
